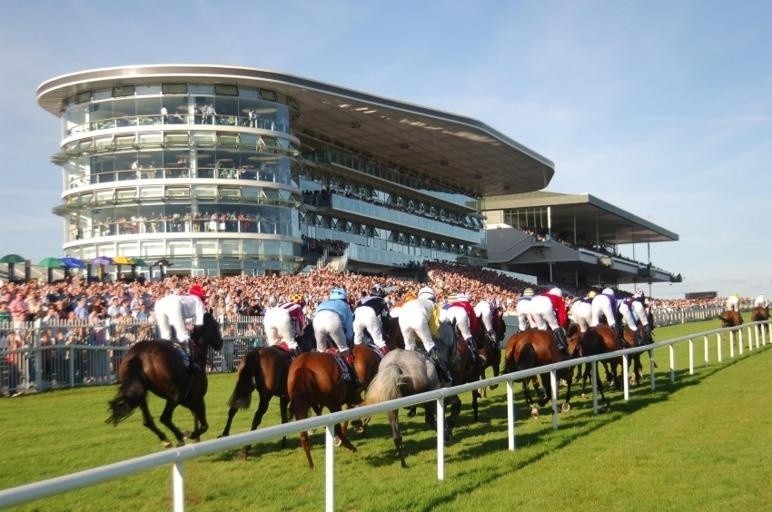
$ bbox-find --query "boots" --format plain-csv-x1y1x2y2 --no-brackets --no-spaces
466,336,479,362
633,329,644,345
429,346,453,382
487,328,499,346
340,349,362,387
184,339,202,368
646,324,655,343
612,324,626,346
554,327,568,350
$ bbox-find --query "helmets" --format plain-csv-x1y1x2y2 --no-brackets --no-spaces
370,285,384,297
587,290,595,298
637,289,644,294
291,293,307,308
447,292,471,306
602,288,614,295
330,288,346,299
549,287,563,298
524,288,533,297
418,288,436,299
189,286,206,302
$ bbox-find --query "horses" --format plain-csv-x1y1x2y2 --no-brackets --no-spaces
104,306,224,449
750,306,770,334
218,304,655,470
718,308,743,346
